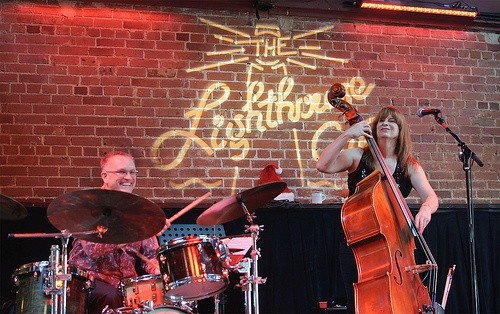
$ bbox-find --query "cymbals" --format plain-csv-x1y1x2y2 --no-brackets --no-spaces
0,192,29,221
195,181,288,226
46,188,166,245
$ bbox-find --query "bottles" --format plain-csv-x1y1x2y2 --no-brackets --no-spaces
320,301,327,314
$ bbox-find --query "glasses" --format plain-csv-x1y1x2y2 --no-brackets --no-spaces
106,169,138,178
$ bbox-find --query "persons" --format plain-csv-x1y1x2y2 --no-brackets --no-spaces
314,104,439,314
67,151,171,314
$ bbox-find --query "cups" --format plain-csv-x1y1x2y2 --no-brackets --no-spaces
312,192,326,204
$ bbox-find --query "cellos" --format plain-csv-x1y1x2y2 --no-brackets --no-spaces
327,81,439,314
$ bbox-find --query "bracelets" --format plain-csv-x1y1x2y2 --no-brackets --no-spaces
421,203,432,213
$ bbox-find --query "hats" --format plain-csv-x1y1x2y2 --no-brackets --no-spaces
258,163,294,202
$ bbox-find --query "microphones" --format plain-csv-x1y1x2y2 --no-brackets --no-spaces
417,108,439,117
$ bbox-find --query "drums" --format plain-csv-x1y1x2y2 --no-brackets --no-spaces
10,259,94,314
101,300,197,314
154,234,230,303
118,272,166,307
